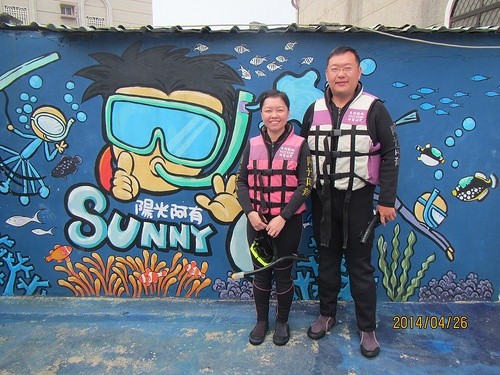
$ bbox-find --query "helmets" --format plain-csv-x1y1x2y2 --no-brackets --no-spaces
250,237,277,268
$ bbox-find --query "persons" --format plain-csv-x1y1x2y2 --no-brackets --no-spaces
300,47,401,358
237,90,314,346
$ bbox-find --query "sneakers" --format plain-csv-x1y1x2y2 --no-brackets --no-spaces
359,330,380,357
273,319,290,345
249,318,269,344
307,314,336,338
